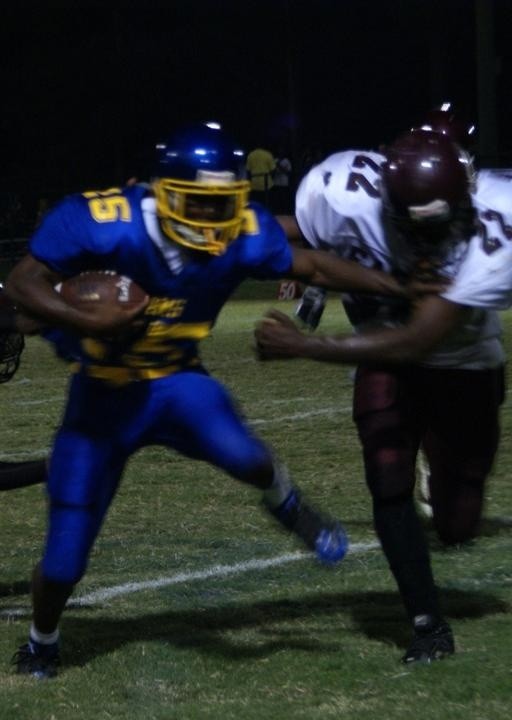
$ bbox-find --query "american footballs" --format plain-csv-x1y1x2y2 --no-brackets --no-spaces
51,269,146,313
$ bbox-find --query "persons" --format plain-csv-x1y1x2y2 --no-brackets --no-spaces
2,123,456,677
243,134,275,208
251,128,512,669
269,138,293,212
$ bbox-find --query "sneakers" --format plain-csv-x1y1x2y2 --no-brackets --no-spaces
265,486,349,562
17,635,64,680
414,446,434,520
402,613,456,664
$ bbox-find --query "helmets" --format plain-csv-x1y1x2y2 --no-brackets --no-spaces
150,122,255,257
382,128,479,257
0,284,25,383
